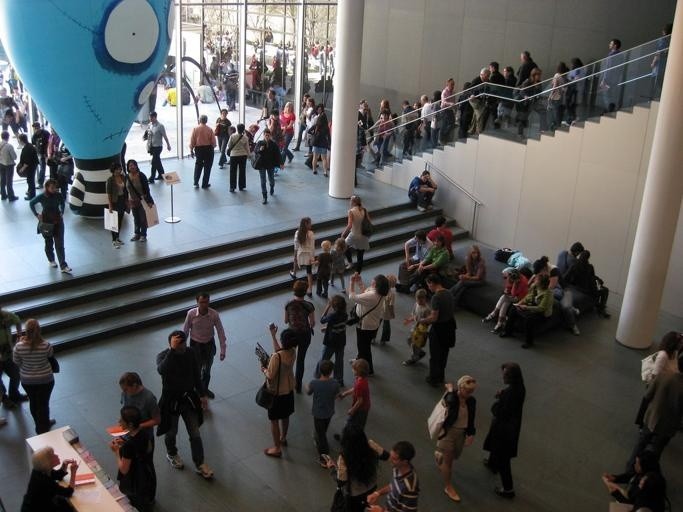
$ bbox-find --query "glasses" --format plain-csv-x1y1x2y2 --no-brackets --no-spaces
460,378,476,385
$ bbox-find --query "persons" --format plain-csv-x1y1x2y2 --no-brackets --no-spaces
190,23,335,204
30,178,72,273
408,170,437,211
356,91,442,167
546,57,585,130
600,39,622,113
1,62,74,213
19,448,78,510
261,196,610,512
106,159,154,248
1,309,57,433
144,112,171,184
442,50,541,144
651,22,672,99
604,330,683,512
113,371,161,510
158,294,227,478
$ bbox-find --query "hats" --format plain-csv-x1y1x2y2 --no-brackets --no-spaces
148,111,157,116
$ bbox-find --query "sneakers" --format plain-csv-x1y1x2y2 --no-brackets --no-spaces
219,162,226,169
194,183,210,188
131,234,146,242
428,377,445,387
482,312,508,338
435,451,461,501
316,280,345,297
147,177,163,182
0,394,28,425
483,458,515,498
207,390,214,399
24,191,32,200
112,238,124,249
402,351,425,367
348,359,357,363
522,341,533,349
571,306,580,336
35,184,43,189
416,204,432,212
195,464,213,479
166,453,184,468
50,418,55,424
409,284,422,292
61,266,72,273
229,188,242,192
289,271,312,297
48,260,58,267
346,265,353,270
371,338,386,345
288,149,328,176
1,194,19,200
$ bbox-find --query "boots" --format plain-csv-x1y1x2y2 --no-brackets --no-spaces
262,184,274,204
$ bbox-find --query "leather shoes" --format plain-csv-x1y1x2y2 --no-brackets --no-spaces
264,434,287,457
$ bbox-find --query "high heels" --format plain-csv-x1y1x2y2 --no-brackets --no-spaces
593,306,610,318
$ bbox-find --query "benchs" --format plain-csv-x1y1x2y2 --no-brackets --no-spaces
398,259,595,337
26,425,140,512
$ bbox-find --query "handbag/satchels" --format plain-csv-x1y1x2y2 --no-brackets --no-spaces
310,133,319,146
17,163,30,177
331,490,350,512
227,149,230,156
214,127,220,136
411,321,427,348
250,151,264,170
346,304,359,326
148,142,152,153
427,399,448,440
257,383,277,410
362,217,374,236
40,222,55,237
496,250,515,263
642,355,656,382
